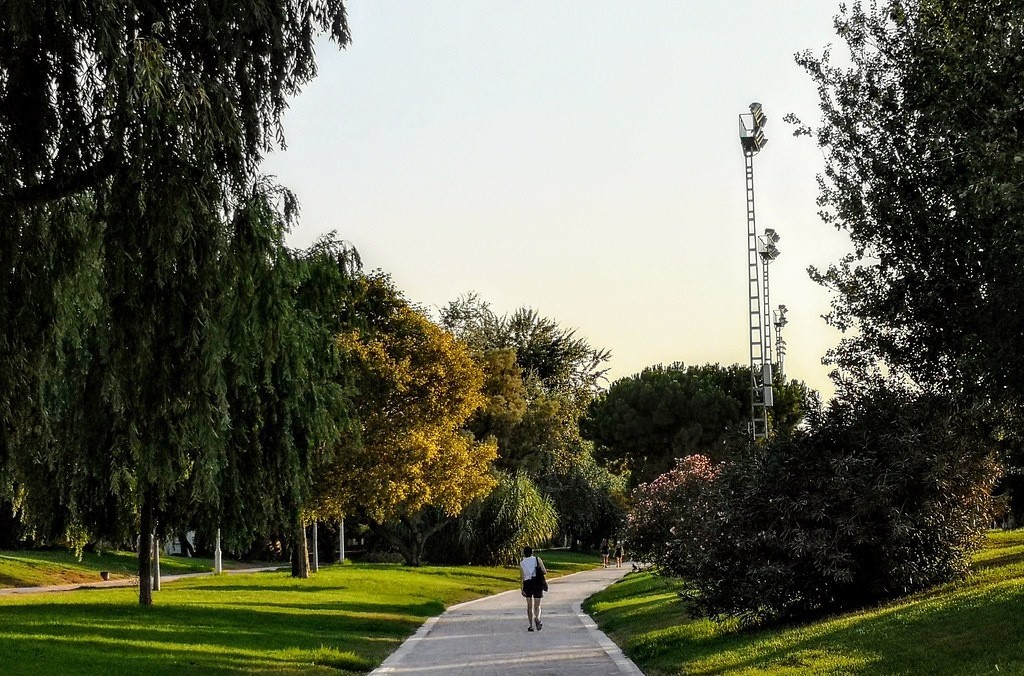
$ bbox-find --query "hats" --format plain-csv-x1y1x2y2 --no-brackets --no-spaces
617,541,621,543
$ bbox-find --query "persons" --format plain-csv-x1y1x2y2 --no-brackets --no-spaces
601,539,610,568
613,541,624,568
521,546,547,631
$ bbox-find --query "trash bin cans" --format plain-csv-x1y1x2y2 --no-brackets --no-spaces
100,572,109,581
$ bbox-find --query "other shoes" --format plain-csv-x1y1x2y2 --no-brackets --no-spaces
528,627,534,631
535,619,542,630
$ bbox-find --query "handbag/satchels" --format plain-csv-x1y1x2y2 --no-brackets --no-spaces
536,556,548,591
